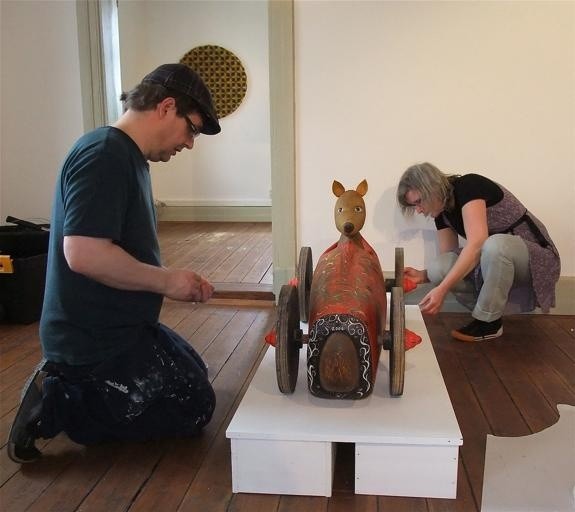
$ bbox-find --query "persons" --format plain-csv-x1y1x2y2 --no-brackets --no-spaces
396,163,561,343
7,63,221,464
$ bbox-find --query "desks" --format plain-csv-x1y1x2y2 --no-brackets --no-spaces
224,302,466,502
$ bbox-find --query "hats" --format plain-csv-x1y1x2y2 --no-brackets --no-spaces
142,64,221,135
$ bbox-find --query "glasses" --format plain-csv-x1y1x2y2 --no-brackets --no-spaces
182,112,202,138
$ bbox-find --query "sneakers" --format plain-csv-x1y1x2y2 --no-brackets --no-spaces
450,319,504,342
7,359,62,463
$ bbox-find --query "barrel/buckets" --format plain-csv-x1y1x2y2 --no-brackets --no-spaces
0,226,51,324
